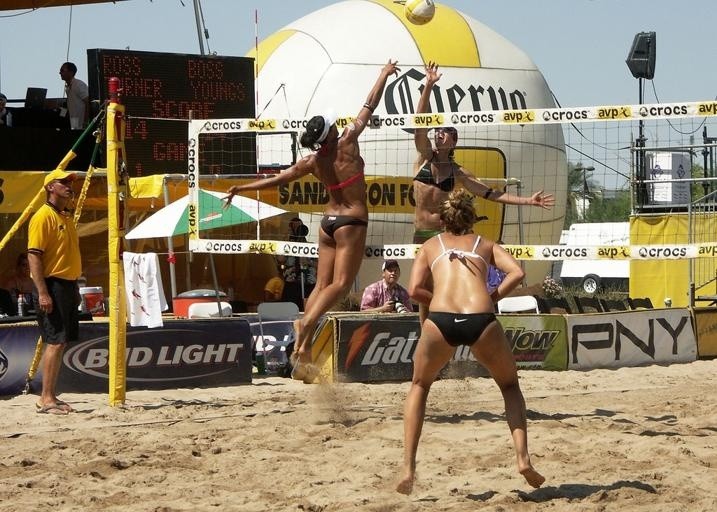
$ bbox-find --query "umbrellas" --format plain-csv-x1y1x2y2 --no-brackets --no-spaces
125,188,291,319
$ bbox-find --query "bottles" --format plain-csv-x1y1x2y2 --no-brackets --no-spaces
16,294,29,318
395,302,406,313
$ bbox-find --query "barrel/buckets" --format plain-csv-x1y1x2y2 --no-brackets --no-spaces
79,286,105,315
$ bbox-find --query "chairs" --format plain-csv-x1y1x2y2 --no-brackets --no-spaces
497,296,539,315
257,301,299,376
542,294,653,314
188,302,232,319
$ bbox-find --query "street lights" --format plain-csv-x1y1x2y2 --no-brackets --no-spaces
573,166,596,222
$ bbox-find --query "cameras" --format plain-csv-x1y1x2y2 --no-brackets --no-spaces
294,225,309,242
389,292,408,314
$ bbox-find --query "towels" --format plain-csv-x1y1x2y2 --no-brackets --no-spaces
122,251,169,326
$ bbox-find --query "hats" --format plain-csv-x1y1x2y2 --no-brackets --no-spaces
382,260,399,272
312,111,339,144
43,167,78,188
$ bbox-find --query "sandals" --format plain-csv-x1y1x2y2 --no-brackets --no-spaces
34,400,76,415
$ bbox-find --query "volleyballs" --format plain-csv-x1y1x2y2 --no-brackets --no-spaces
404,0,434,23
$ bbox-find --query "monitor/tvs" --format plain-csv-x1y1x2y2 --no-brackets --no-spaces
24,87,47,110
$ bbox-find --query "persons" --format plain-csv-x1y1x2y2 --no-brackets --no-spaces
27,169,82,415
263,218,318,312
396,187,545,495
59,62,89,130
360,260,413,312
413,61,556,326
0,94,12,127
5,253,32,293
220,58,402,353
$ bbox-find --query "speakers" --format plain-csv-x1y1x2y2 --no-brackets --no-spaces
626,30,656,79
